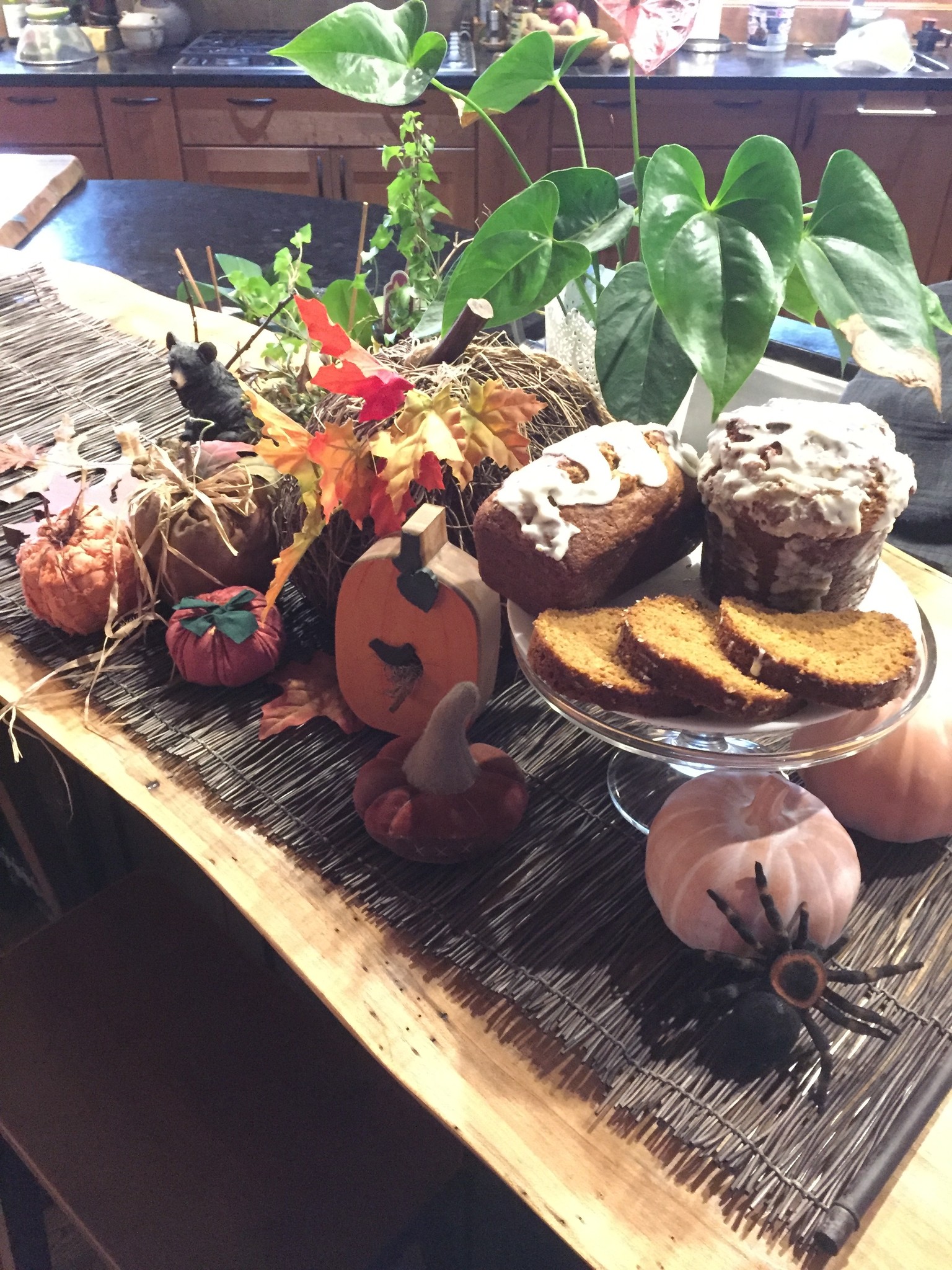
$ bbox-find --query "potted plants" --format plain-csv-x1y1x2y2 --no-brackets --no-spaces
268,1,947,446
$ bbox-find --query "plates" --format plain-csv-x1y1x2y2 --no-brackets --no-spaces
507,543,937,838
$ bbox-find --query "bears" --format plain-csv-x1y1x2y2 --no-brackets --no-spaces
166,331,265,458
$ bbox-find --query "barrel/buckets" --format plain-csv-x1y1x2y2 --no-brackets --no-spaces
746,2,796,52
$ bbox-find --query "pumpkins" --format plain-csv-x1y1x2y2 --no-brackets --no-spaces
644,697,951,959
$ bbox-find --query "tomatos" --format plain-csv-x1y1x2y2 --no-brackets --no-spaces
548,2,578,26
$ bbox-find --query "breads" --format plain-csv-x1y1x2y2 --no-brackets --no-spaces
473,397,921,724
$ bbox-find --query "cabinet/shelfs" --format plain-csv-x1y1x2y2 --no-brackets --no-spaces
562,80,797,270
799,86,950,288
89,76,186,184
3,75,109,182
176,76,475,236
476,82,555,236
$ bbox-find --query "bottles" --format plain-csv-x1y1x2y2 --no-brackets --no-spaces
479,10,508,50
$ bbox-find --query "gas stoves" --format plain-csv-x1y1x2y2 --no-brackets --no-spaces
172,26,478,77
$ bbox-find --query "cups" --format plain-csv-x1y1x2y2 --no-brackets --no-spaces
117,11,165,56
